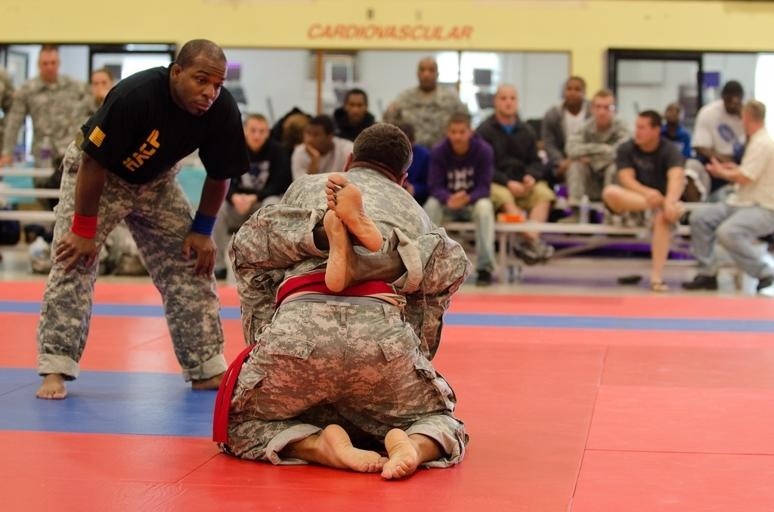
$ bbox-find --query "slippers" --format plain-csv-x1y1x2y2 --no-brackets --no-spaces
650,281,669,292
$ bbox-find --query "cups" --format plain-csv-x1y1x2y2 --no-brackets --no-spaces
502,264,522,281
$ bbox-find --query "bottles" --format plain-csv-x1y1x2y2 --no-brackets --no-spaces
38,136,51,167
580,195,591,223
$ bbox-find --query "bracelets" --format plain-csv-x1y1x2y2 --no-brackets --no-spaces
69,211,97,239
190,211,217,237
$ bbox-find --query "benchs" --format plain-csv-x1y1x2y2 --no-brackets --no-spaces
1,167,748,292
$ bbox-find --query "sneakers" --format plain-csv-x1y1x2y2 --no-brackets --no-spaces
683,275,717,289
514,237,555,265
477,272,491,285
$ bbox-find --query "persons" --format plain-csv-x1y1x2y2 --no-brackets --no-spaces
211,59,773,291
1,44,144,275
32,39,243,401
229,175,468,364
211,122,466,480
